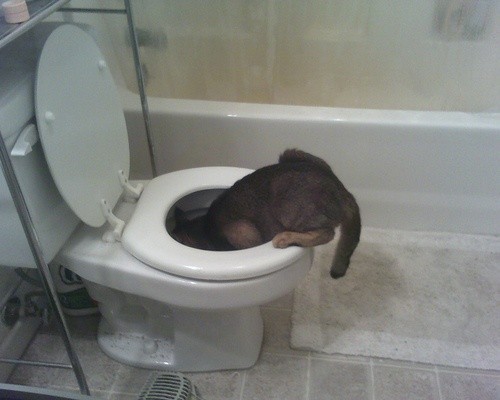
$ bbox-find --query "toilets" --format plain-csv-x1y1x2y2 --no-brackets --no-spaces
0,23,315,374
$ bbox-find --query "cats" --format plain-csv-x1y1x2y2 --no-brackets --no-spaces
170,146,363,280
